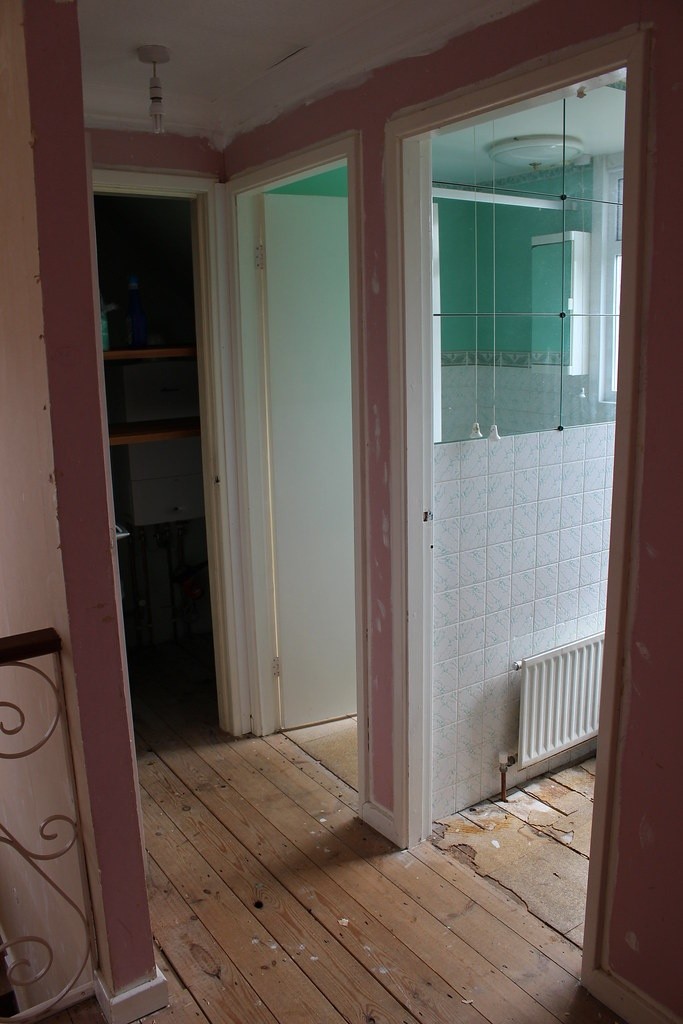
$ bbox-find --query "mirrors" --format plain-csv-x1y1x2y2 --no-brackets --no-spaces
428,79,626,443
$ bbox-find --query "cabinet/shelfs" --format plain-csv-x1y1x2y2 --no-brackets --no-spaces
108,359,204,527
104,344,200,445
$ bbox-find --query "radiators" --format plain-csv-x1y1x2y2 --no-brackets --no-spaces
498,632,605,803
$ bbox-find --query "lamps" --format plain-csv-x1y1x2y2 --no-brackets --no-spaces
490,134,585,168
